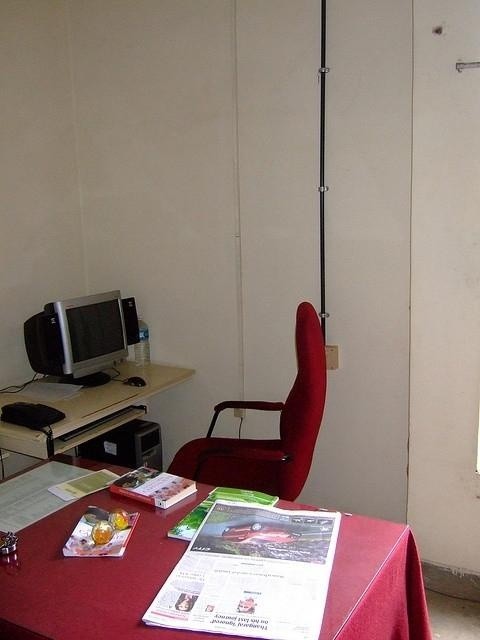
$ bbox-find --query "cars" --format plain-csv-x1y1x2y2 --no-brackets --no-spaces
222,524,301,543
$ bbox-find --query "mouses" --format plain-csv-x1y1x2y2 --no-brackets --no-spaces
125,376,146,388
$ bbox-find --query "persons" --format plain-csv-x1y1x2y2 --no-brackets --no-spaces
238,595,259,613
177,594,197,611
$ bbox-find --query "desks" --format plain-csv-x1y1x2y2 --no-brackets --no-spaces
0,447,438,640
2,359,199,460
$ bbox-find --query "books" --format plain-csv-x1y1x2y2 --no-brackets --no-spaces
109,466,198,509
142,500,341,640
63,506,140,556
167,486,279,542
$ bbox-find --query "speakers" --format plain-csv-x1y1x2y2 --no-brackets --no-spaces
122,296,142,346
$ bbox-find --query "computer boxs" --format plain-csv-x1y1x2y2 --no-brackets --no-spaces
79,418,163,471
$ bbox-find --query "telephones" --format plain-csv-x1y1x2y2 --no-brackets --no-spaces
1,401,67,431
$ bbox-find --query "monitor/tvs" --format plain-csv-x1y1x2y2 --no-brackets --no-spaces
23,288,129,390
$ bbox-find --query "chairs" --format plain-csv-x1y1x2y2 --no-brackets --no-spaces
164,303,326,501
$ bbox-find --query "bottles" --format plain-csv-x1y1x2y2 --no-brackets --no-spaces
134,314,151,368
